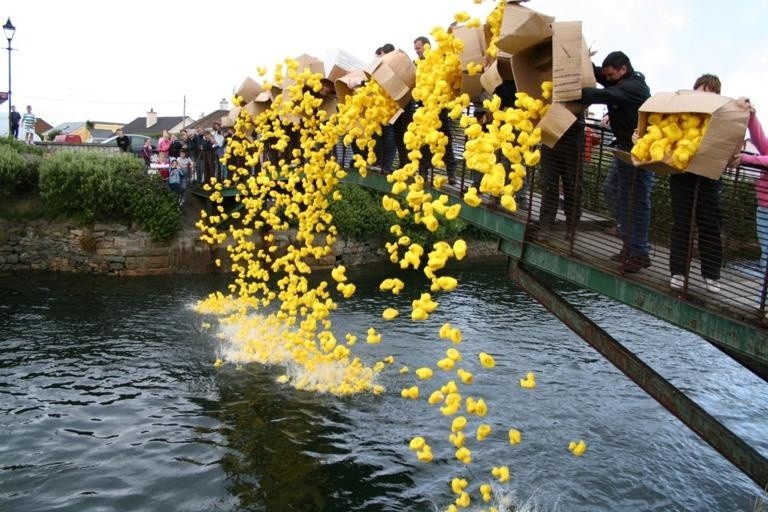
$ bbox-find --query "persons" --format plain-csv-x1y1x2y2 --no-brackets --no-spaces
9,105,21,138
567,53,654,273
729,96,768,318
601,112,619,236
631,74,723,293
21,104,37,145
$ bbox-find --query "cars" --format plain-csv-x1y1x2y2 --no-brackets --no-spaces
98,134,162,151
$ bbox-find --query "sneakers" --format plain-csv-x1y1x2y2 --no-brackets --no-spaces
669,274,686,291
448,176,457,185
599,220,620,238
609,244,652,277
380,168,394,175
704,277,721,293
463,182,579,241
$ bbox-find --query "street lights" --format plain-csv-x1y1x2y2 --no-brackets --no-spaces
2,15,19,141
